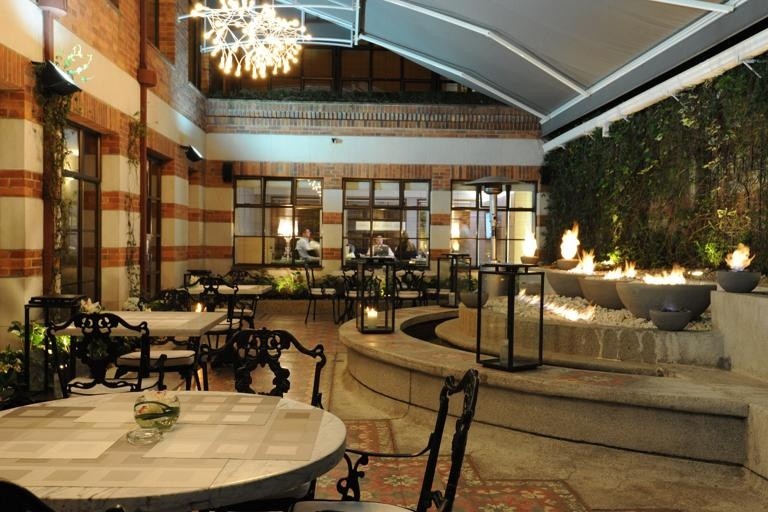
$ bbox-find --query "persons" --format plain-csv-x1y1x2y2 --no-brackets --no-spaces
343,237,355,258
367,234,394,258
395,233,417,260
295,228,320,270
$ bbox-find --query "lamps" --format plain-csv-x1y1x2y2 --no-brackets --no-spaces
38,59,82,96
185,144,204,162
190,0,311,80
